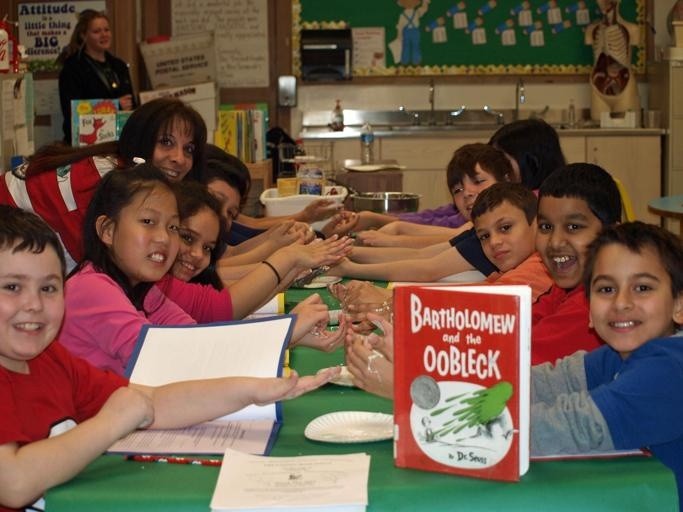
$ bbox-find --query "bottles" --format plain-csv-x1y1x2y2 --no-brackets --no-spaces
330,99,344,131
359,121,375,164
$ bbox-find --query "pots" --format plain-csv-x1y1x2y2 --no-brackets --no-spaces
329,175,424,215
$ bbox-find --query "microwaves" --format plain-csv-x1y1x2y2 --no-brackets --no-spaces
300,27,352,82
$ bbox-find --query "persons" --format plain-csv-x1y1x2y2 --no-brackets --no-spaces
321,118,623,366
0,205,339,510
1,99,208,278
153,143,355,325
343,219,682,511
56,9,135,144
53,162,345,381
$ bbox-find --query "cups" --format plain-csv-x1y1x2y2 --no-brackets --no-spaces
643,110,660,128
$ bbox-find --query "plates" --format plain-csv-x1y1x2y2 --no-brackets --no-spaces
409,380,515,469
320,365,353,388
304,409,393,445
301,273,341,290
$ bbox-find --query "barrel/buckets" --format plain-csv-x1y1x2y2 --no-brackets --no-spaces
259,184,350,289
351,189,423,212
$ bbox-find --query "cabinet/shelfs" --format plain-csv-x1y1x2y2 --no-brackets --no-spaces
558,129,585,165
646,60,682,242
300,130,379,177
586,130,662,230
380,131,492,214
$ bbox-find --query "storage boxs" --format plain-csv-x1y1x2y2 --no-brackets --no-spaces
136,26,220,88
258,185,347,229
139,81,221,132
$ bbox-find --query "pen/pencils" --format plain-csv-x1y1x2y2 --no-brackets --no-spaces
126,454,223,465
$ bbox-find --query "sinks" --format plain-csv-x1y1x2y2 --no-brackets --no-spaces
388,119,503,133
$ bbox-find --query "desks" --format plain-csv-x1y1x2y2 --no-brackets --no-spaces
645,194,682,234
47,275,679,512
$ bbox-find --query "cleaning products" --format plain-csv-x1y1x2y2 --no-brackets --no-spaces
292,155,325,196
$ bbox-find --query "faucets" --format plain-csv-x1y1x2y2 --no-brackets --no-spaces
514,77,526,111
428,78,436,116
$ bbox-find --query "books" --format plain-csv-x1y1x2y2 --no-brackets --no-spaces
102,316,294,454
391,284,533,482
